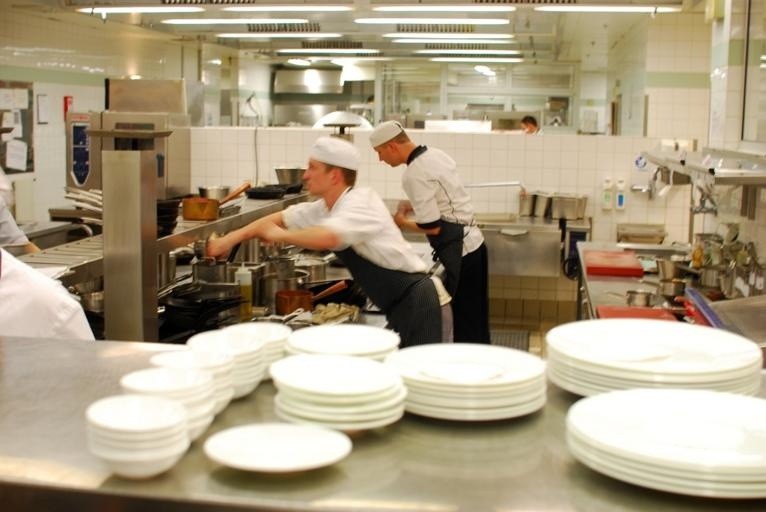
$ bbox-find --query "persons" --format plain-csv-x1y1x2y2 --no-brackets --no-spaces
8,268,95,345
519,113,543,135
205,133,453,350
369,119,491,347
1,198,41,256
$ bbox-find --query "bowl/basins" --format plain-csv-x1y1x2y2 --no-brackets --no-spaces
81,319,293,480
72,275,104,314
197,185,230,200
274,168,307,182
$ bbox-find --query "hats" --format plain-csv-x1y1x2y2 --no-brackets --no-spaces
308,136,360,172
368,120,405,148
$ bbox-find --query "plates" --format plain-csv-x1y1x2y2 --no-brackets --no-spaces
203,419,355,478
383,340,548,422
563,387,766,502
545,317,765,398
266,322,408,439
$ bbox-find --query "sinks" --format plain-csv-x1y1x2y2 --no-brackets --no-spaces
622,246,690,263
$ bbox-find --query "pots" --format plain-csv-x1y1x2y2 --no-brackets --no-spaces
47,185,181,236
636,278,686,296
181,181,252,220
265,183,304,194
603,289,654,309
156,233,339,325
633,222,759,282
243,186,285,200
595,305,679,321
273,279,348,315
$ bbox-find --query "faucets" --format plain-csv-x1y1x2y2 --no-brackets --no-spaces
689,193,718,217
463,182,526,196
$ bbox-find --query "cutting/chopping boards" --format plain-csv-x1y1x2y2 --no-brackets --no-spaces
582,250,644,277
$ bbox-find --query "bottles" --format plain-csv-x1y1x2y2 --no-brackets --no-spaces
233,261,253,321
